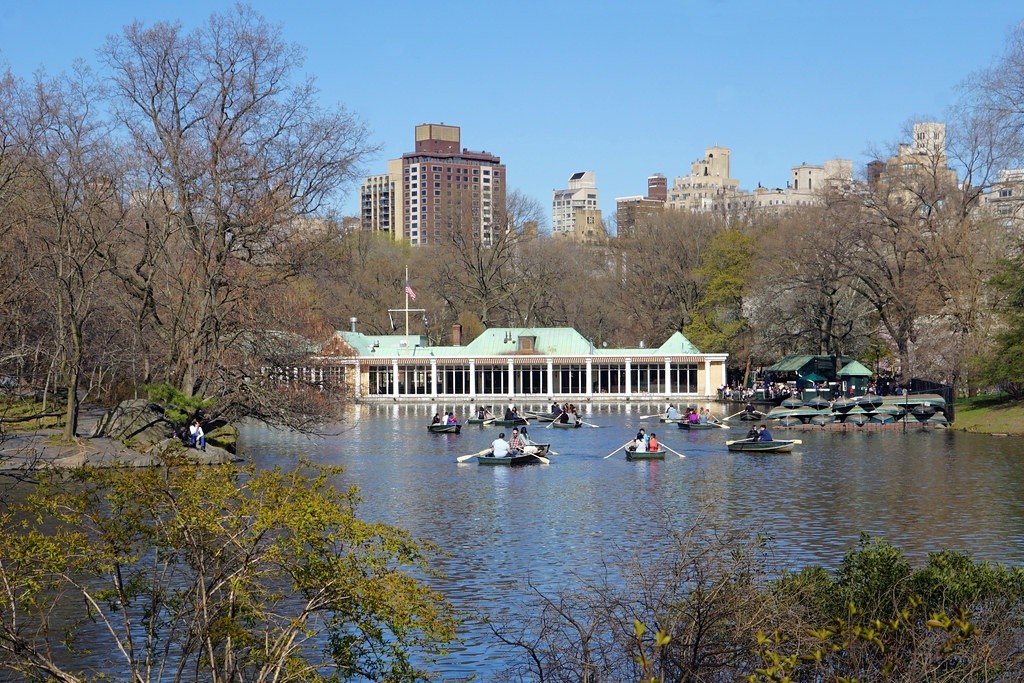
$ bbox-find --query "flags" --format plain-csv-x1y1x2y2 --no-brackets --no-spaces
405,282,416,301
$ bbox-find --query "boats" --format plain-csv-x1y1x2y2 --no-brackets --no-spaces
740,411,762,421
677,421,713,431
728,440,795,453
764,394,949,428
495,411,584,429
660,414,686,424
624,441,667,461
476,442,551,466
468,417,495,424
430,423,462,434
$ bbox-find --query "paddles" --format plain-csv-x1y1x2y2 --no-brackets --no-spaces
456,448,494,463
517,412,531,426
602,435,637,460
575,420,600,428
528,439,559,455
483,415,505,425
525,412,553,421
755,410,767,416
546,412,563,429
707,421,731,430
639,413,665,420
722,410,746,421
772,438,803,445
726,437,754,446
712,415,720,422
664,419,683,424
647,435,686,458
485,408,499,422
516,446,550,464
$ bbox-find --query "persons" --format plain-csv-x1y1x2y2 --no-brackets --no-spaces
635,428,657,452
492,427,530,458
816,373,903,396
505,407,520,420
478,406,485,419
666,403,711,424
720,381,797,400
746,425,772,441
432,412,458,426
190,422,205,451
745,401,755,413
552,402,577,423
